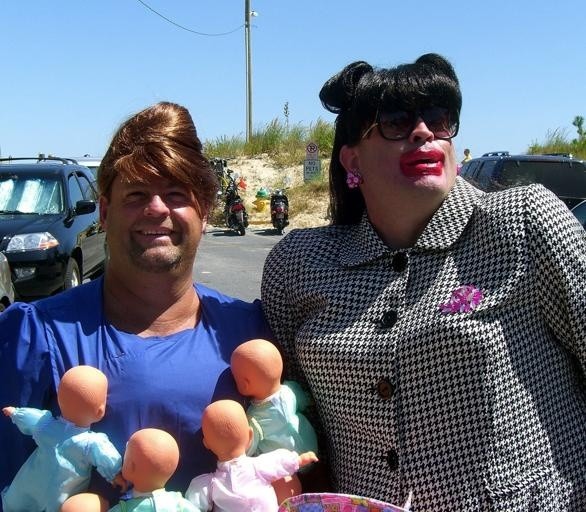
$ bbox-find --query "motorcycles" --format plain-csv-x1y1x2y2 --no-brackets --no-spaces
218,181,249,236
271,189,290,235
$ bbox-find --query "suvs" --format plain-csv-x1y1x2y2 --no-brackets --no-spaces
0,157,102,311
461,150,585,211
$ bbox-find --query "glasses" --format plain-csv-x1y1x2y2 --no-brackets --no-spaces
362,105,460,141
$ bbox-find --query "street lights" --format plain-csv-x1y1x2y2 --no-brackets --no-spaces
245,11,258,145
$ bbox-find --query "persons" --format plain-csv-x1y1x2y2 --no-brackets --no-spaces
0,338,318,512
0,101,267,512
1,338,318,512
461,148,473,166
262,50,585,511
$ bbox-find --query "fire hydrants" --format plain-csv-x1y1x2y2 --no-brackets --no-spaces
253,189,268,211
